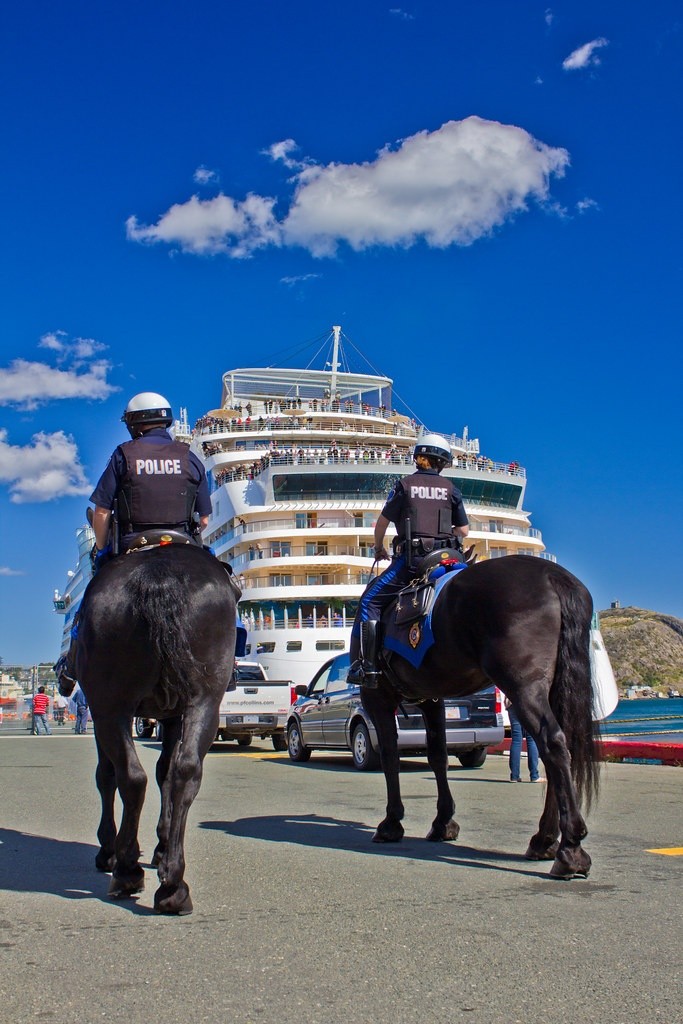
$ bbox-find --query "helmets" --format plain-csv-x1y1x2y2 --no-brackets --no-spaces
120,392,173,426
413,434,452,463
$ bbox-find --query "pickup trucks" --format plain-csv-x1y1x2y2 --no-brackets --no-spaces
217,661,298,752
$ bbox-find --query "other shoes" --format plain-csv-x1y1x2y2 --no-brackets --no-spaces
530,777,548,783
510,778,521,782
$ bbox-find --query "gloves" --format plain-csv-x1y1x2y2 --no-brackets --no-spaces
90,537,113,575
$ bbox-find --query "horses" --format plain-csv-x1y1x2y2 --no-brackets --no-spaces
58,539,237,915
349,544,602,880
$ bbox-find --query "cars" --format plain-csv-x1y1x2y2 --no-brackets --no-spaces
283,649,503,772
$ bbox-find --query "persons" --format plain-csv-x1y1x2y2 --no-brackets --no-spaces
246,575,254,588
195,397,519,482
503,695,549,783
243,613,342,630
228,552,235,567
54,392,212,698
239,574,246,589
56,695,69,726
238,518,247,534
248,544,255,561
72,689,89,735
255,541,264,559
215,524,233,542
348,436,471,689
31,686,52,736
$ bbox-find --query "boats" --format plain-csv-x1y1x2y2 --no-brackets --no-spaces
53,325,621,738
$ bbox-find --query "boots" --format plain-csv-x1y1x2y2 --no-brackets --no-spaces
346,620,381,689
54,612,80,698
